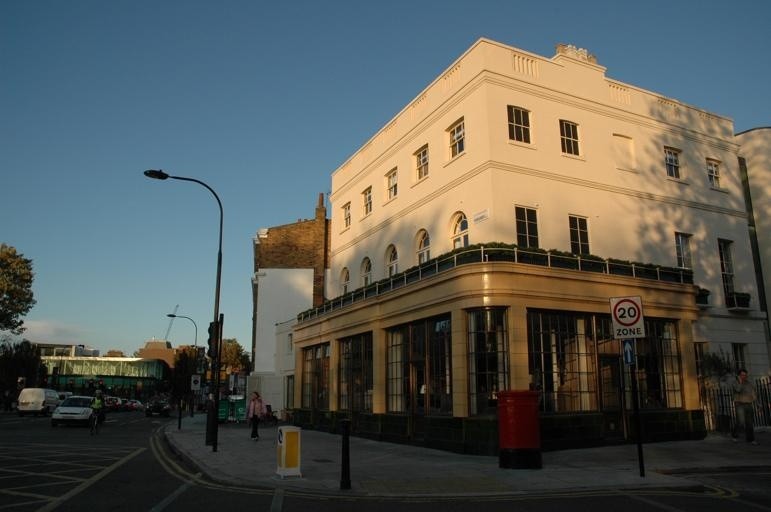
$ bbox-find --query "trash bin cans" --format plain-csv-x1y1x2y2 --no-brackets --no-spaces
276,426,302,480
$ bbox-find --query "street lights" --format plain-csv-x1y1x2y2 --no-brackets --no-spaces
58,344,84,390
166,314,198,417
145,166,224,452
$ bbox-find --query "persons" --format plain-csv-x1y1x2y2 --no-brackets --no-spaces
728,368,761,446
245,391,268,440
87,390,107,432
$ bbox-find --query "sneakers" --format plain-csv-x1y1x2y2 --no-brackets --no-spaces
251,435,260,442
731,437,760,447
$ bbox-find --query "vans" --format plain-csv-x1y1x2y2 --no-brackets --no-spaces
17,387,59,416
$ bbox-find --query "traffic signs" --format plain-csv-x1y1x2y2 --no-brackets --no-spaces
611,295,645,339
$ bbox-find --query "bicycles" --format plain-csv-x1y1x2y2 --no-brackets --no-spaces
89,406,98,436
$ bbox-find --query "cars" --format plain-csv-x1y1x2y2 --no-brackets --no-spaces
51,396,94,424
104,397,144,411
146,397,168,416
